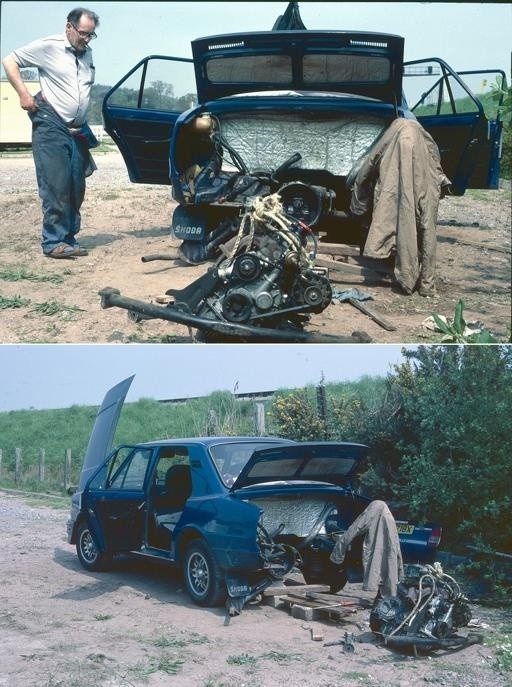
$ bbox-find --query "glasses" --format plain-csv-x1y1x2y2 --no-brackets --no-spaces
69,20,97,40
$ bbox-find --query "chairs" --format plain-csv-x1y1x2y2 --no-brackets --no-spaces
157,463,191,509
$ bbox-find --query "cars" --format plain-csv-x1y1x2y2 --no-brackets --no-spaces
96,0,511,265
63,373,383,614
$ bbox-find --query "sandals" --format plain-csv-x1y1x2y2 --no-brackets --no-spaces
69,240,89,257
43,243,77,257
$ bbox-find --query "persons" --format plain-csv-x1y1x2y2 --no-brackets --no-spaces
0,7,102,259
